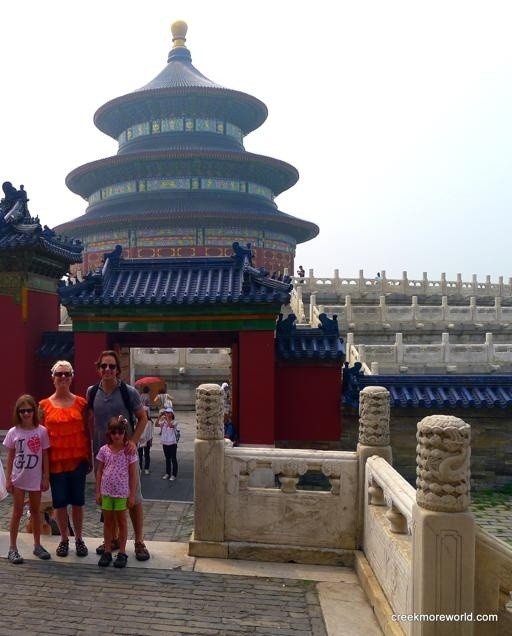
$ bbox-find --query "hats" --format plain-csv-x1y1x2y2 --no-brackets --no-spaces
165,407,173,413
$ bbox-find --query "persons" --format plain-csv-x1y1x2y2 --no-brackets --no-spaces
95,414,138,569
154,387,174,437
140,385,153,412
374,272,381,284
224,414,239,447
154,406,179,482
222,382,229,401
134,405,155,477
86,349,150,562
296,265,306,285
2,394,52,565
37,359,95,559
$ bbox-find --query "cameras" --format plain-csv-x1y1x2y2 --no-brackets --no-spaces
161,411,166,416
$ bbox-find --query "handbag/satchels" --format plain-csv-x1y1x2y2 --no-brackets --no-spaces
164,394,172,408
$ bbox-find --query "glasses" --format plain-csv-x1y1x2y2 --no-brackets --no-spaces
18,408,33,414
110,430,124,435
98,363,116,369
53,372,72,378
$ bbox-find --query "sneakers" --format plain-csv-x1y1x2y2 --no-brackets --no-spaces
169,475,176,481
8,550,23,564
56,540,69,557
32,546,51,560
135,541,150,561
144,469,150,475
75,541,88,557
98,551,112,567
96,538,121,555
162,473,169,480
113,552,128,568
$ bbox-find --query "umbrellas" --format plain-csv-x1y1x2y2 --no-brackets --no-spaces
133,375,162,386
